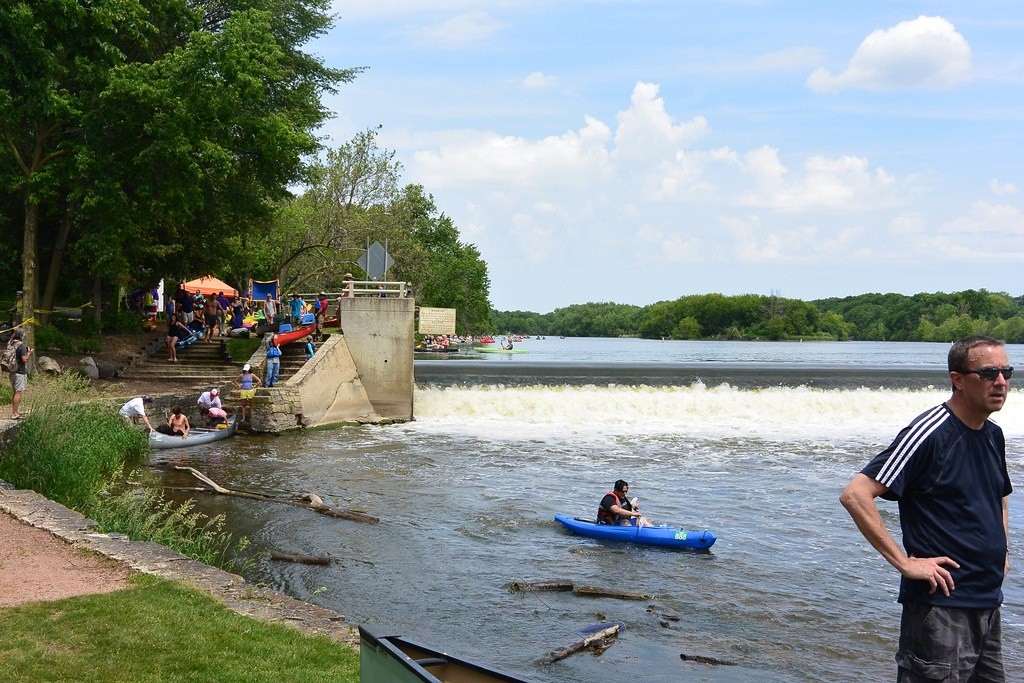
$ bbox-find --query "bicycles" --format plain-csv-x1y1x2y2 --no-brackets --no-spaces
0,311,14,342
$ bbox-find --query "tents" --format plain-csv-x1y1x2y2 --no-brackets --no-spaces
178,274,239,298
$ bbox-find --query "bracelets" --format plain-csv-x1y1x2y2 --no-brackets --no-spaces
1006,548,1009,552
185,433,188,435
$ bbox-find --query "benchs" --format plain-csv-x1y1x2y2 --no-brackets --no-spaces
188,427,220,434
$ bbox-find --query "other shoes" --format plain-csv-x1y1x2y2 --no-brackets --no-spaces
167,358,173,360
174,359,177,361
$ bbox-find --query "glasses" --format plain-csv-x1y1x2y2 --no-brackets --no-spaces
620,490,627,493
957,366,1015,382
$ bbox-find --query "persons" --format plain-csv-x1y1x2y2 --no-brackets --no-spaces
159,407,191,439
118,395,156,432
197,389,223,428
201,408,229,431
839,334,1015,683
9,328,33,419
265,332,282,387
6,290,23,326
234,363,261,422
120,272,356,363
596,480,655,527
421,333,563,350
377,285,389,298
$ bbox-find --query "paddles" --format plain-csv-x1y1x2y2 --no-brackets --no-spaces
630,497,646,531
501,341,504,350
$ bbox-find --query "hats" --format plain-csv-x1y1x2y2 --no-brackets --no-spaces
344,273,354,278
307,335,312,341
320,292,326,295
242,364,249,370
210,389,218,396
14,327,24,337
291,292,299,296
266,293,272,297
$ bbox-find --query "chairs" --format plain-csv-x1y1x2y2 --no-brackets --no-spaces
279,324,292,333
302,313,315,325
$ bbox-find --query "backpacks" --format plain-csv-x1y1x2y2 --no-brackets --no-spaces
0,338,24,373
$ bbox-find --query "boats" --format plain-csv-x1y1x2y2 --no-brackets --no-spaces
148,415,237,449
358,623,533,683
176,317,207,348
554,513,717,550
480,337,495,343
142,320,157,331
473,347,528,353
324,315,338,327
414,347,459,352
273,320,316,347
513,338,522,342
